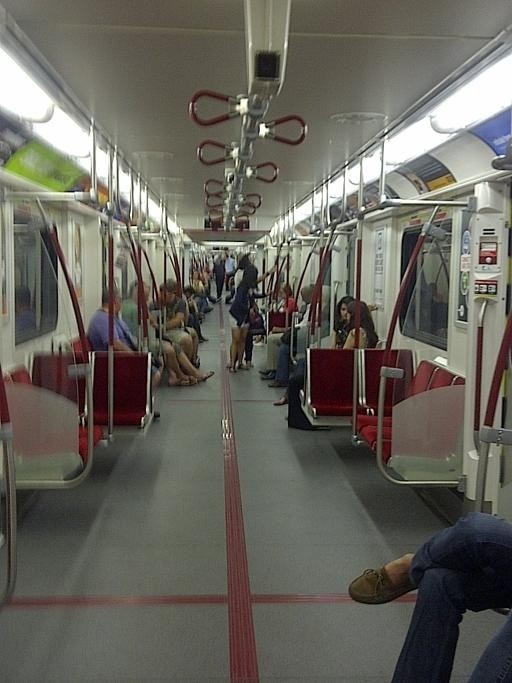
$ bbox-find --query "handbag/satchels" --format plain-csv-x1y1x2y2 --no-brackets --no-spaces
230,298,247,322
248,308,266,336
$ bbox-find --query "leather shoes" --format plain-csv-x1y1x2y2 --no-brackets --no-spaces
348,565,418,605
260,368,275,380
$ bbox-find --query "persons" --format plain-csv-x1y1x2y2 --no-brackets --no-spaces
348,512,512,683
16,286,36,331
469,611,511,683
88,272,215,418
428,283,443,306
258,284,377,407
415,304,437,334
203,254,277,373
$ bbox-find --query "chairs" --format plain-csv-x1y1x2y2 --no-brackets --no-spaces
301,348,361,428
1,329,154,490
354,357,475,486
363,347,415,404
